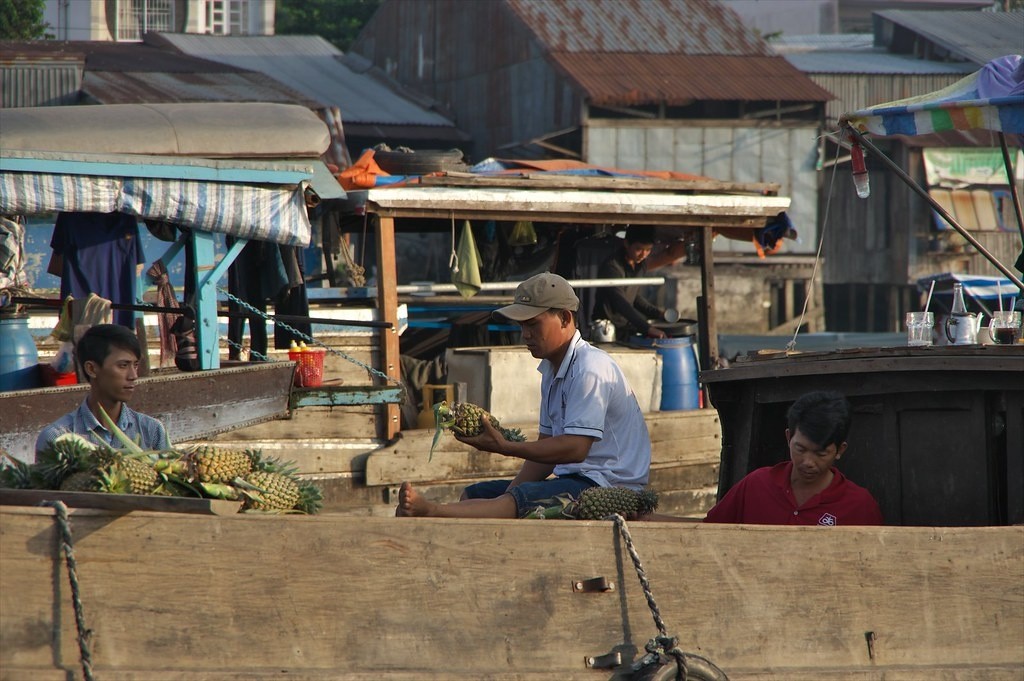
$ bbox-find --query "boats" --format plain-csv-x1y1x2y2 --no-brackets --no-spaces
9,61,1024,681
0,101,406,469
50,148,804,519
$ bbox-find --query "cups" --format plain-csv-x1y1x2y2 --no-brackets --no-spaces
906,311,935,347
988,310,1022,345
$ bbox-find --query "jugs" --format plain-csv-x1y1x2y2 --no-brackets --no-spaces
946,312,985,346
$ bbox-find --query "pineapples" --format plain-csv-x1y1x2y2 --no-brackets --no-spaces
524,487,661,520
430,401,528,443
0,433,322,514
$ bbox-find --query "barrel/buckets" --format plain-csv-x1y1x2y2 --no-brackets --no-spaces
0,318,40,393
633,317,703,412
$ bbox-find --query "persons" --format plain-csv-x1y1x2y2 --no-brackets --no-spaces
225,233,289,363
645,389,886,525
394,272,651,519
590,223,668,339
35,323,172,466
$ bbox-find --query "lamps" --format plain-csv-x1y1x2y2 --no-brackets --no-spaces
849,144,871,198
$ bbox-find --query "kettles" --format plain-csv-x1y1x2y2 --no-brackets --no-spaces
591,319,617,343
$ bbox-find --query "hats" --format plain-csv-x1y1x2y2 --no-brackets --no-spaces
493,270,579,321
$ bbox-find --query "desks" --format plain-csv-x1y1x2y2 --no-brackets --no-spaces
914,272,1024,326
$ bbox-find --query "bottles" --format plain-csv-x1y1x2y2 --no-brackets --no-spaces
950,282,968,314
290,339,319,377
